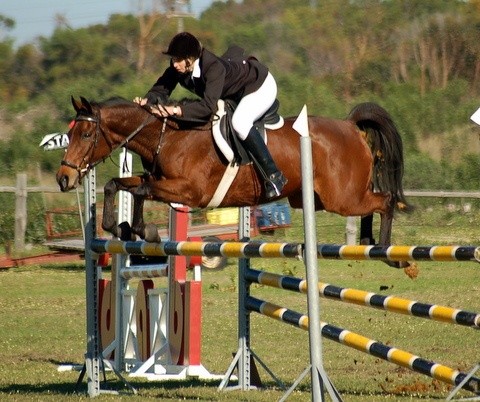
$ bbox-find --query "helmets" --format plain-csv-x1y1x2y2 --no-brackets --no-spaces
163,31,201,58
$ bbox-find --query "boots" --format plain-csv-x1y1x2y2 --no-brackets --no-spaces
243,125,287,198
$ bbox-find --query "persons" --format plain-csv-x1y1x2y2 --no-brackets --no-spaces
132,31,288,201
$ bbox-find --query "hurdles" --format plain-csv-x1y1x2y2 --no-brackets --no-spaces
82,136,480,402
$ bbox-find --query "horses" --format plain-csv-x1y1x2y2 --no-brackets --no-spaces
55,95,414,269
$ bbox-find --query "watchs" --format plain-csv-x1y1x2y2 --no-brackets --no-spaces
172,105,178,116
